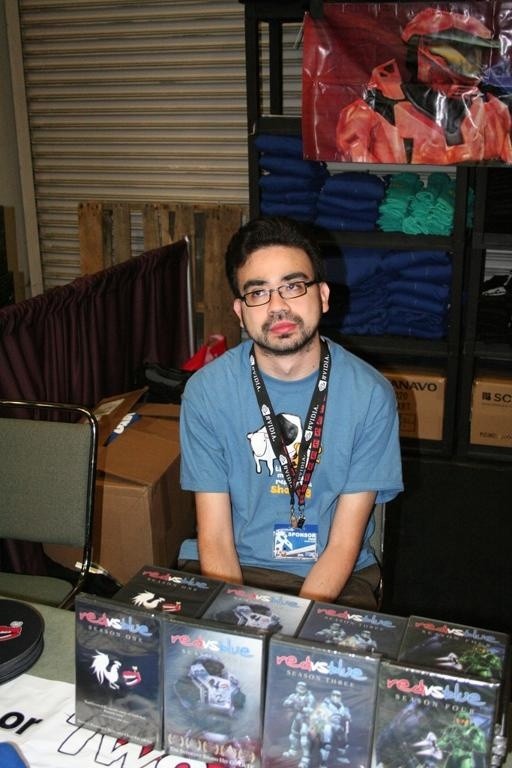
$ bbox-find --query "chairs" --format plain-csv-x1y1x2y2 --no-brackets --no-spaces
0,398,98,610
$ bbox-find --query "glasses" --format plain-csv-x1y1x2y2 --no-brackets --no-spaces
241,279,316,307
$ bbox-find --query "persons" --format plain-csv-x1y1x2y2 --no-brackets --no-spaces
175,214,407,614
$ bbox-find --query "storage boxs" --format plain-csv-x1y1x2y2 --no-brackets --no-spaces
45,386,197,587
369,363,511,449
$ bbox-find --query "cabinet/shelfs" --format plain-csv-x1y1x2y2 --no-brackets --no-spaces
240,0,512,465
